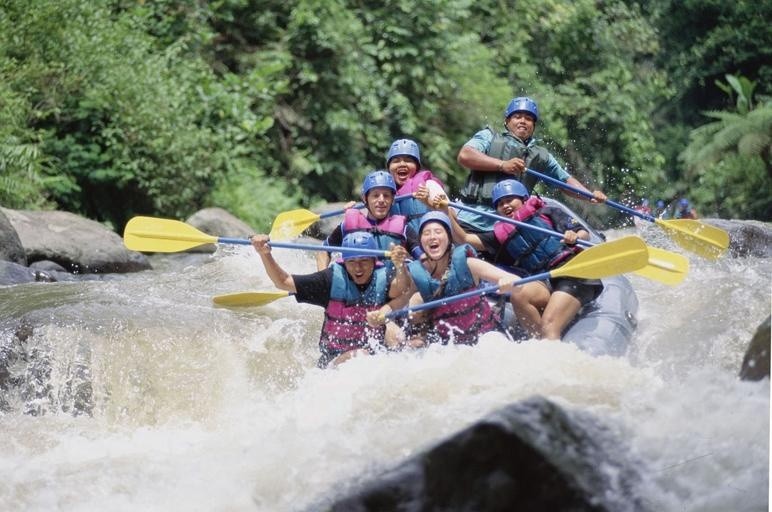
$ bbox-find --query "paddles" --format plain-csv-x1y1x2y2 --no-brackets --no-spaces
521,166,730,262
438,199,688,287
124,216,393,258
378,234,648,320
213,291,299,308
269,192,417,242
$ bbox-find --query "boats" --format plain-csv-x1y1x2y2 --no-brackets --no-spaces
496,188,641,359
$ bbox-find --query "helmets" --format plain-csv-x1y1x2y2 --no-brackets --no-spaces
418,211,453,239
342,231,377,259
387,139,420,164
363,170,396,194
506,96,537,122
492,179,529,210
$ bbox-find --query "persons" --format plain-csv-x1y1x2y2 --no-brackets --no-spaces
250,232,412,371
343,139,453,234
366,211,524,346
433,179,604,339
314,171,428,272
447,96,608,262
